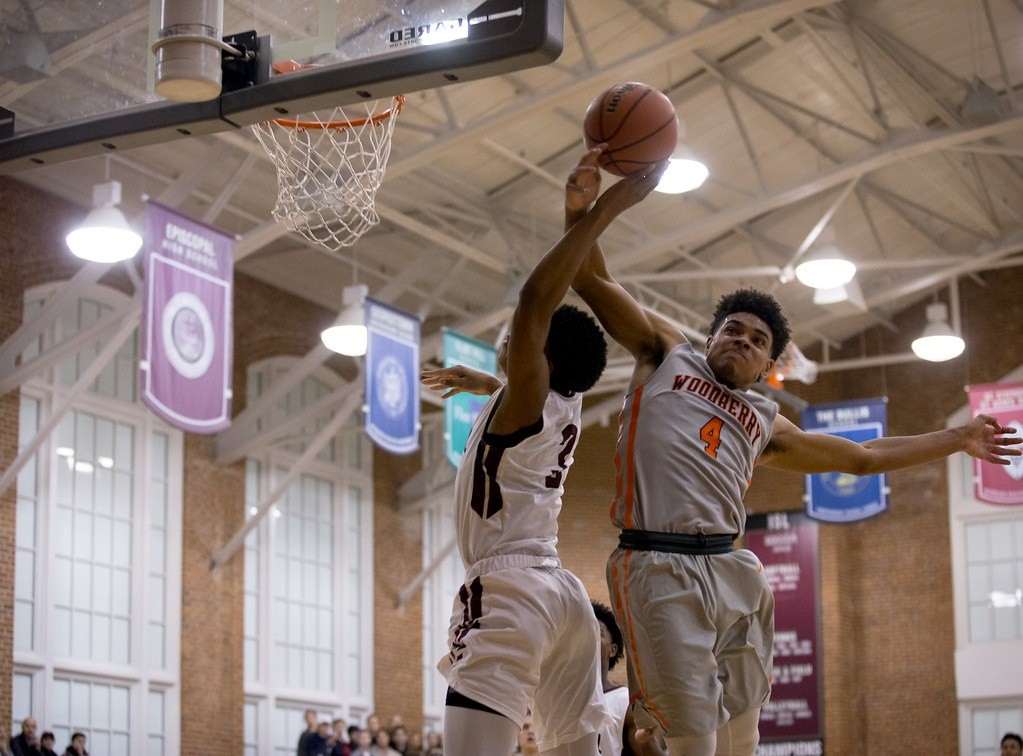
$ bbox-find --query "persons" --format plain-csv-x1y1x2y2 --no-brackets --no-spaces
1000,733,1023,756
513,708,540,756
297,708,443,756
419,161,670,756
10,716,92,756
564,145,1023,756
591,600,670,756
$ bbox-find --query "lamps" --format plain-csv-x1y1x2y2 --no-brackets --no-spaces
793,240,856,289
911,292,967,361
654,8,708,195
65,156,142,262
318,249,368,356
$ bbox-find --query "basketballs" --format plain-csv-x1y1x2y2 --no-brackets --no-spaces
582,81,680,177
